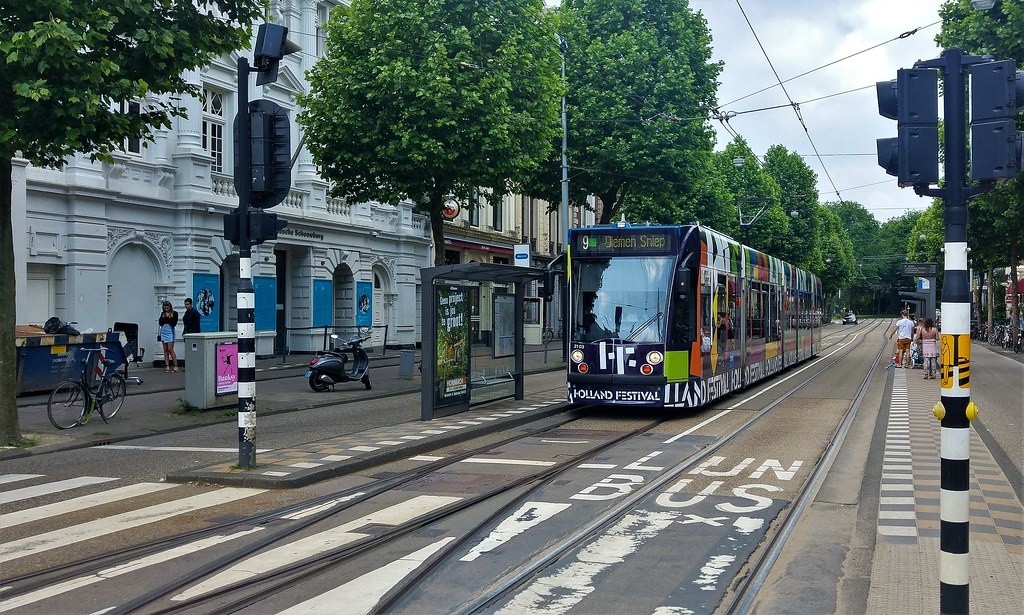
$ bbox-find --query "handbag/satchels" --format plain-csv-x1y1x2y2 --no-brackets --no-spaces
157,336,161,341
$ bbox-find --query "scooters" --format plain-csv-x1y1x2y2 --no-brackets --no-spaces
305,324,373,392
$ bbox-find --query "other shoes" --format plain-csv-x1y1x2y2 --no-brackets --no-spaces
929,375,936,379
924,374,928,379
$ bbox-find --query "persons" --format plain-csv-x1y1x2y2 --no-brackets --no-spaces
158,301,180,374
697,309,737,353
889,310,915,368
908,314,940,380
182,298,201,342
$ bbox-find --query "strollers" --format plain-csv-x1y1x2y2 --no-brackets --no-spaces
909,342,924,368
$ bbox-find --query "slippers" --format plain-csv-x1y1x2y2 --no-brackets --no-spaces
904,366,908,368
895,366,901,368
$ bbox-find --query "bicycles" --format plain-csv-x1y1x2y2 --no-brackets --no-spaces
47,347,127,430
543,323,554,345
558,328,564,339
971,321,1024,354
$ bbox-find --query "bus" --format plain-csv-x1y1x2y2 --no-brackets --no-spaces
544,198,823,409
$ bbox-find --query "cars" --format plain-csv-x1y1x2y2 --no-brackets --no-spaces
842,313,858,325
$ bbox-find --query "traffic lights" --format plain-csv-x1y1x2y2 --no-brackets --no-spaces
970,57,1024,179
254,22,303,69
249,212,287,240
876,67,939,187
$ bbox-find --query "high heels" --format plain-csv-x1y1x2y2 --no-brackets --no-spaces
171,365,179,373
165,365,170,372
